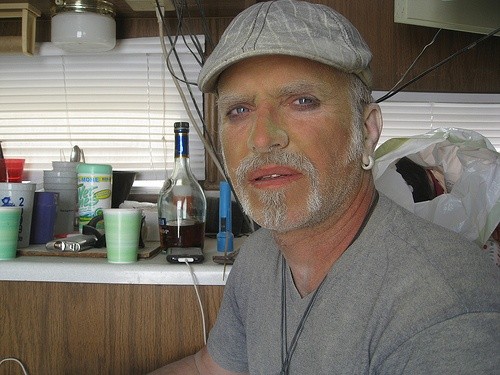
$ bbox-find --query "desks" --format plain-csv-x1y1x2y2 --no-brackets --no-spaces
0,236,244,375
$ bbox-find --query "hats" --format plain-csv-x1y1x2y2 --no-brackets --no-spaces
197,0,378,94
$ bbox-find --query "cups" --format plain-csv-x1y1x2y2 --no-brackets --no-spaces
43,161,82,233
30,192,60,244
0,207,22,261
102,208,143,264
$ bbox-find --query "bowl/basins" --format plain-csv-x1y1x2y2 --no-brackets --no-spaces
0,159,25,183
112,170,136,209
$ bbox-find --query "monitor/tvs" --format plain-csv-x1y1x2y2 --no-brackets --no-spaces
393,0,500,36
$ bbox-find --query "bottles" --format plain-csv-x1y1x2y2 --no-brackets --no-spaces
158,122,206,256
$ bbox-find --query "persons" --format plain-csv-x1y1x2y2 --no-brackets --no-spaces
143,0,500,375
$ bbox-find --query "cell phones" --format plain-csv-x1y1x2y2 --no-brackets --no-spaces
166,247,204,264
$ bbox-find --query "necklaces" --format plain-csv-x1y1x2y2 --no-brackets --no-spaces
279,189,381,375
